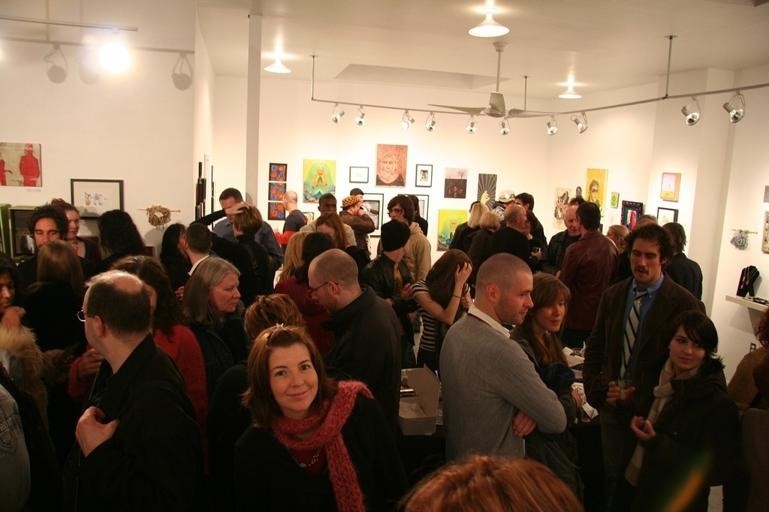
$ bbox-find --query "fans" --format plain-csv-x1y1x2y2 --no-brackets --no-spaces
427,39,551,119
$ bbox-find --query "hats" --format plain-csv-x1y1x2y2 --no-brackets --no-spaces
499,189,515,203
381,220,410,252
340,195,360,208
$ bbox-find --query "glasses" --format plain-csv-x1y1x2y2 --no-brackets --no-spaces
77,310,102,323
307,281,339,294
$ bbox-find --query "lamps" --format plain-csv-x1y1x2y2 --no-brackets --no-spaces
465,114,512,134
402,108,437,132
557,75,584,100
264,41,295,75
468,1,509,37
679,93,745,126
328,101,369,129
545,113,587,133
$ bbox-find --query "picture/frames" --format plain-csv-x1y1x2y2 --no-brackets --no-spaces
415,194,429,221
415,163,433,188
620,200,643,226
69,176,126,218
347,166,369,184
362,192,383,237
657,206,678,227
266,162,287,221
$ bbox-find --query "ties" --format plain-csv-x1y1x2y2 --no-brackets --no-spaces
620,290,646,379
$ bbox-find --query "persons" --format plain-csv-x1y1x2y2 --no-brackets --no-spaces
1,185,768,512
20,144,40,186
379,149,404,184
0,159,12,185
